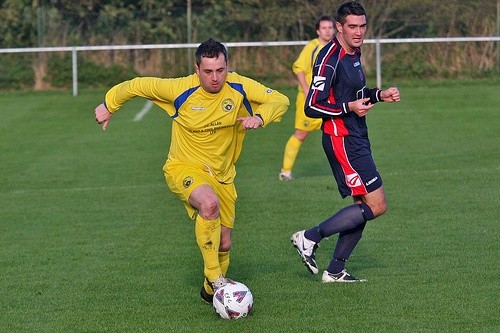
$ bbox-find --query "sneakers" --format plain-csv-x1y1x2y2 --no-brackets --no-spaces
322,264,361,282
279,170,292,182
199,284,214,303
290,228,319,276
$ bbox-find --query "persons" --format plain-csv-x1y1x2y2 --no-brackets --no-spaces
291,1,400,283
95,38,290,305
279,15,334,182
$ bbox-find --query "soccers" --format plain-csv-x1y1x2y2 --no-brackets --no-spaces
212,281,254,319
